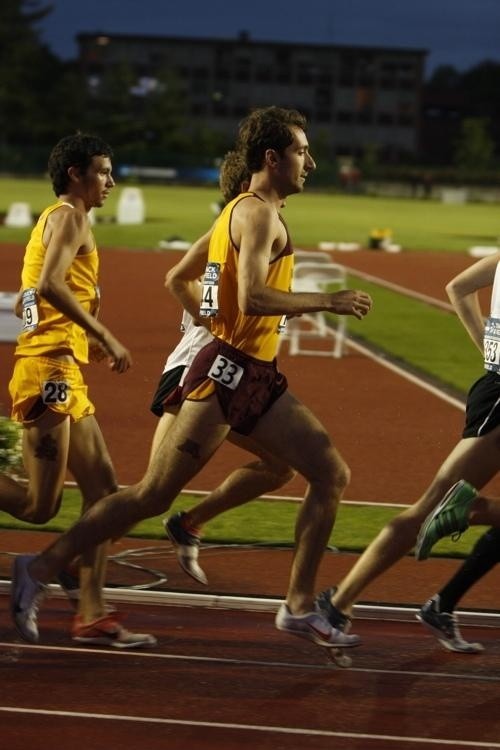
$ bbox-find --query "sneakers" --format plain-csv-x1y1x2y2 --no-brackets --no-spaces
10,555,158,650
162,511,209,585
416,479,480,561
275,586,483,668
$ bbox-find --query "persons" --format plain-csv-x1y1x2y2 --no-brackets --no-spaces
53,149,297,614
12,104,372,648
309,248,498,670
411,477,499,561
1,132,160,652
